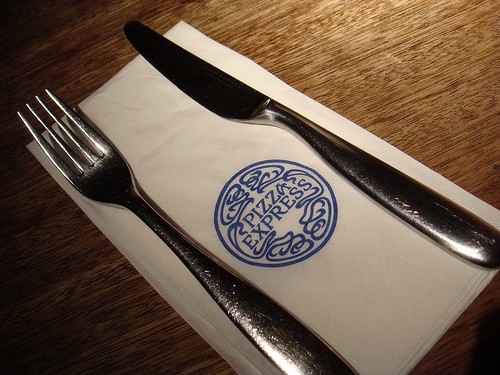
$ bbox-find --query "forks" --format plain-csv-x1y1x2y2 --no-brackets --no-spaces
17,87,357,374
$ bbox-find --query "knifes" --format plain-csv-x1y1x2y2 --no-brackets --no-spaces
123,18,500,268
